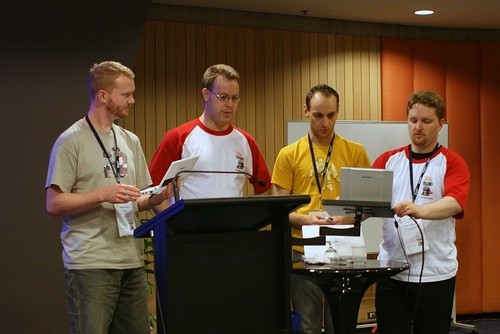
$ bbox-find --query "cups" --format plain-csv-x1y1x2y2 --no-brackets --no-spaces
352,246,366,268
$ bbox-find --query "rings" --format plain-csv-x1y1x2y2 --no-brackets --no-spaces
406,206,409,210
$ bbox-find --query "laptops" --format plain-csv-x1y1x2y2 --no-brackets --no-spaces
339,167,394,203
140,155,200,194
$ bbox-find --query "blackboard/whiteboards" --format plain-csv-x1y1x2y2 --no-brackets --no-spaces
284,119,450,255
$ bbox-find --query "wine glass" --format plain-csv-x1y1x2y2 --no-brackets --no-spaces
323,240,339,270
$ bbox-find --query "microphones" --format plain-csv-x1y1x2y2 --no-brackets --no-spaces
173,170,266,202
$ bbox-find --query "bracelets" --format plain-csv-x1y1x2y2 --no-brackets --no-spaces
149,194,161,206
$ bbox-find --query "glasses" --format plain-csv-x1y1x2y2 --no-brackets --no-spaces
206,86,241,103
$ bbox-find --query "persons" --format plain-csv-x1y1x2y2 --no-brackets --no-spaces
271,85,373,334
44,59,182,334
370,91,472,334
148,64,272,215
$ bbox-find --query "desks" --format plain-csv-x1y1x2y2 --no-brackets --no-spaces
293,260,412,334
